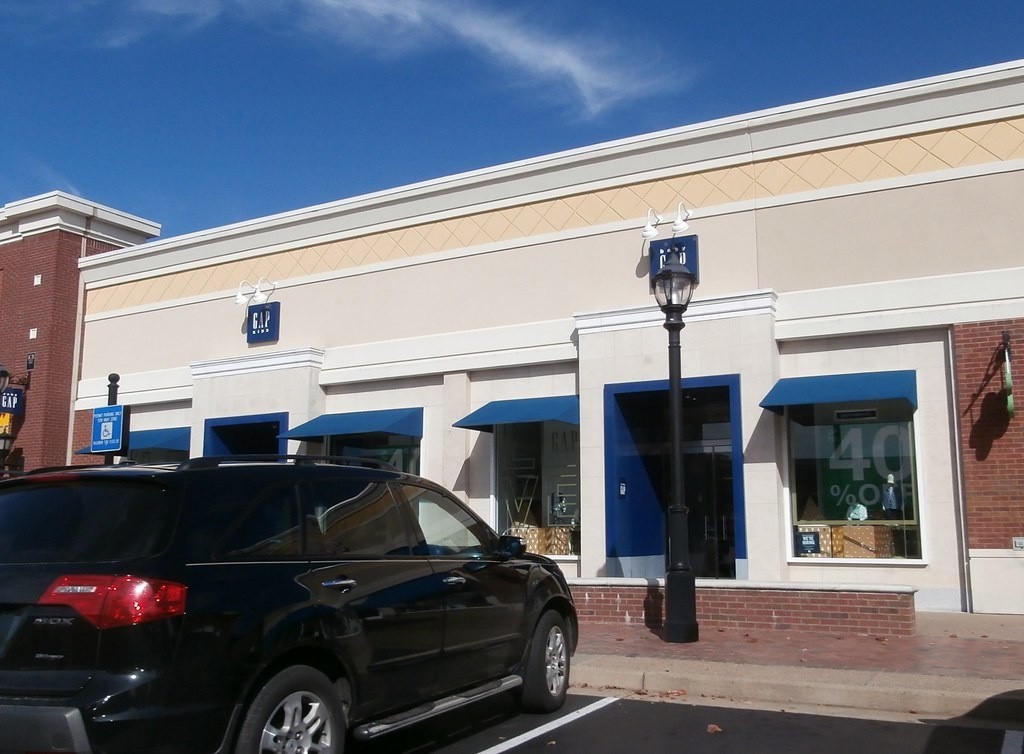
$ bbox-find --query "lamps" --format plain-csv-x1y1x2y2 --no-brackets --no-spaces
672,202,692,233
642,208,664,239
235,280,256,306
253,277,277,302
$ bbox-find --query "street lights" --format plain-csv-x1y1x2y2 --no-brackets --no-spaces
652,240,705,641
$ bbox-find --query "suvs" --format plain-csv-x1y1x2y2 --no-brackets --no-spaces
0,453,579,754
